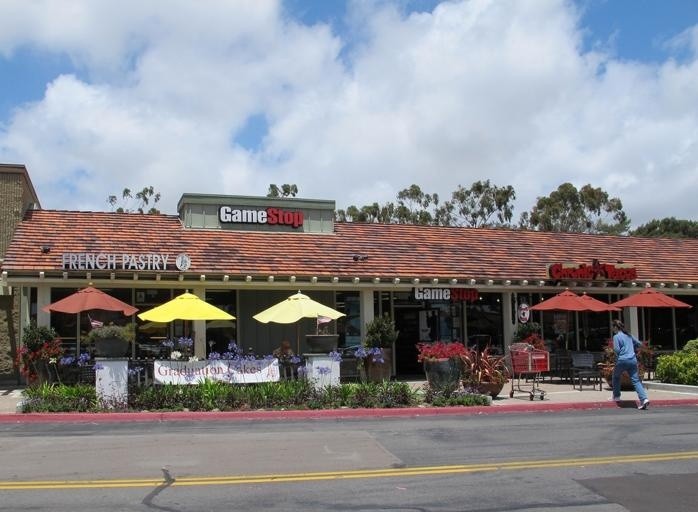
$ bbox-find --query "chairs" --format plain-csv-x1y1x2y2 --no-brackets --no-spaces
551,351,605,390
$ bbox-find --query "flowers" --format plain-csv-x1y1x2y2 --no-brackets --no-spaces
417,342,468,363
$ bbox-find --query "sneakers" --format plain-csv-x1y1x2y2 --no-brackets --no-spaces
637,399,650,410
608,394,622,402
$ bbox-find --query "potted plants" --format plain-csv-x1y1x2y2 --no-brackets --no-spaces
462,344,511,397
80,322,138,357
602,338,656,391
360,312,400,383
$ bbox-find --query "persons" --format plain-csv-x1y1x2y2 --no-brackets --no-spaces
612,318,649,410
274,338,293,358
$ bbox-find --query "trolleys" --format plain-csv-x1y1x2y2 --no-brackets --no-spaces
508,343,550,401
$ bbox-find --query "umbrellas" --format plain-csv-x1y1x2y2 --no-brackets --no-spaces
580,292,623,313
612,284,693,342
252,287,348,354
38,282,139,361
525,286,593,349
135,289,237,336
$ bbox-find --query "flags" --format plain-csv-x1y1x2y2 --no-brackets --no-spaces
90,317,103,328
317,314,332,326
